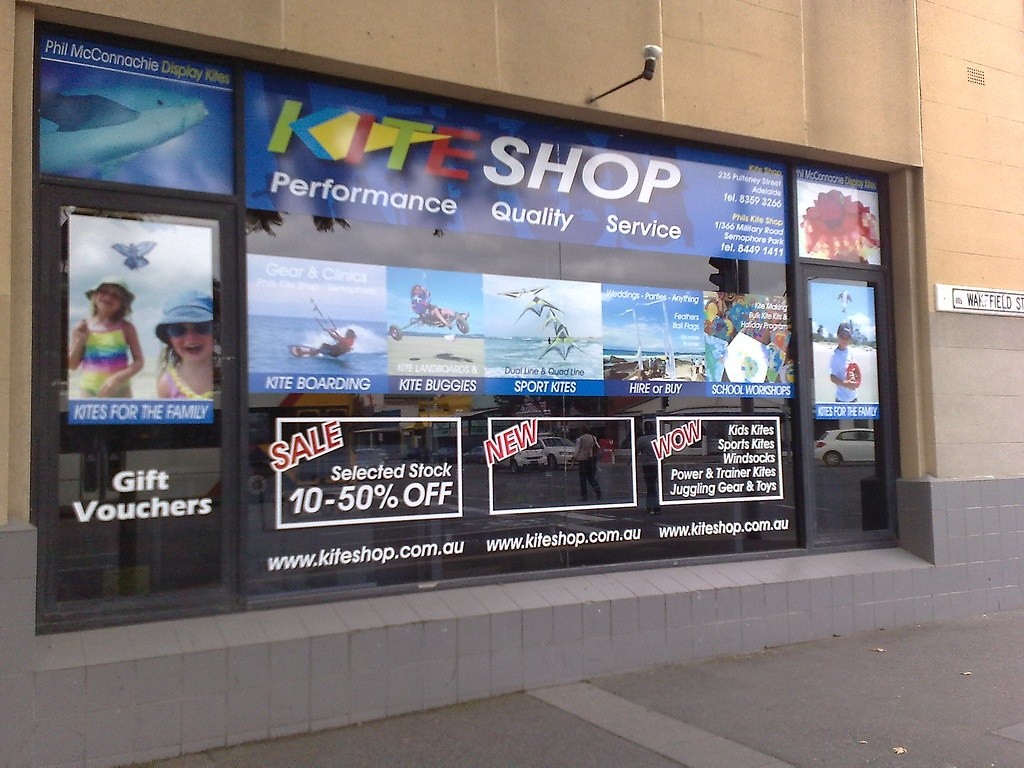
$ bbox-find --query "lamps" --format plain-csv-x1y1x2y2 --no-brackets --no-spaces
588,43,661,106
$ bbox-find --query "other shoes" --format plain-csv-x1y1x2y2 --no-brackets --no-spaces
644,504,661,514
595,488,602,500
578,497,588,502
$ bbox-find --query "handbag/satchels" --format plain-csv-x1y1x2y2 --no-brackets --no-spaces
593,437,600,459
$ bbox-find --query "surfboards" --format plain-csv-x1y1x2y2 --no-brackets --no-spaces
288,344,319,358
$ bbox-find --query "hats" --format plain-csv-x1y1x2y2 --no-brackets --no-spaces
837,322,853,337
155,290,214,341
85,275,136,303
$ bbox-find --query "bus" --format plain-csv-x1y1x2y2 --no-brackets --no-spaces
48,394,362,525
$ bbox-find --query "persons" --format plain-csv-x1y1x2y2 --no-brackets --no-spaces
421,300,454,329
829,322,859,402
154,289,214,400
628,421,662,515
297,329,356,358
69,277,145,397
571,427,602,501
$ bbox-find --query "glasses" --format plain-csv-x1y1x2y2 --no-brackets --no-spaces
93,288,124,303
163,324,212,336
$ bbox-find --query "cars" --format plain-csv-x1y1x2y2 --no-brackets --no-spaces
814,428,876,468
353,424,581,486
